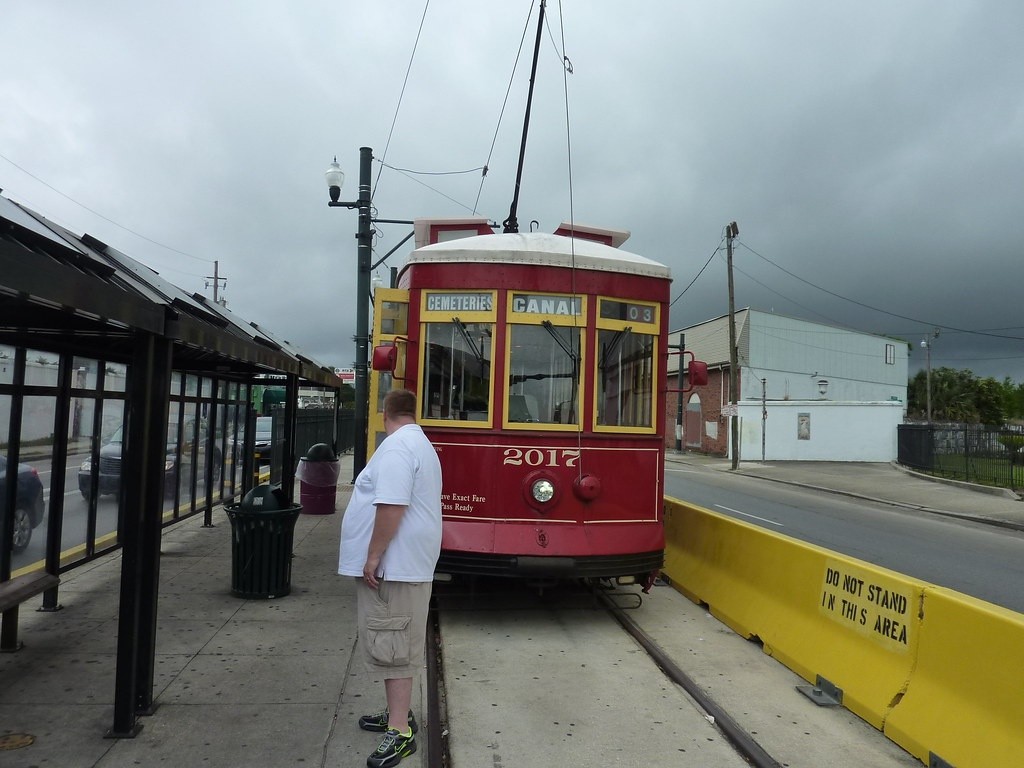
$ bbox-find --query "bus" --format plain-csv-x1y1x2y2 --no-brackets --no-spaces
364,0,707,610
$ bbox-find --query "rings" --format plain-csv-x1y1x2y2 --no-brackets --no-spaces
367,578,371,581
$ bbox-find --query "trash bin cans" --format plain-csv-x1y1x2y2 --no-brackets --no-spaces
223,484,304,600
298,441,341,515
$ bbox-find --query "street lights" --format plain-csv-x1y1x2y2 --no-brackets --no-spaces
324,146,372,484
921,332,932,424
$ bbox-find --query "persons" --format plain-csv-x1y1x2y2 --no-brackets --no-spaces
338,390,442,768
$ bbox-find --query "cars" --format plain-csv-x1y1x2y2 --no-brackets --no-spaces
0,456,45,555
228,417,273,467
77,414,225,499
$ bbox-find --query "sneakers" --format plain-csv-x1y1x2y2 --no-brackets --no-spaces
358,705,419,733
366,727,417,768
